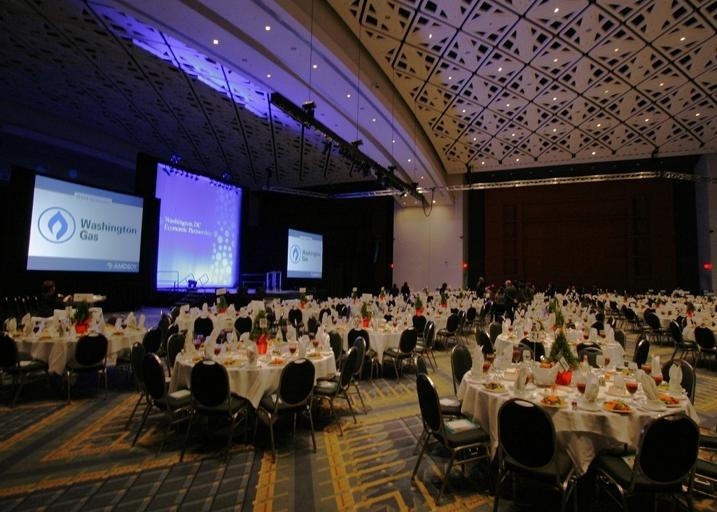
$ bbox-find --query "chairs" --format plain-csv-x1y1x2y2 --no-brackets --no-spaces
476,292,716,370
0,290,149,404
681,432,717,499
593,413,700,510
151,291,494,413
126,347,191,448
182,360,241,462
495,399,576,512
261,358,317,462
412,356,490,505
314,345,357,438
412,375,490,505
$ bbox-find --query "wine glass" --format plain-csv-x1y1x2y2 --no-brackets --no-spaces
480,350,662,405
191,334,320,361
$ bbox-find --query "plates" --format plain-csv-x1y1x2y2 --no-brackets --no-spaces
178,344,334,369
470,366,690,414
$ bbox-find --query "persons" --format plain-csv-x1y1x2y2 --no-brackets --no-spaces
477,277,555,319
400,281,410,302
37,281,71,318
391,283,399,298
